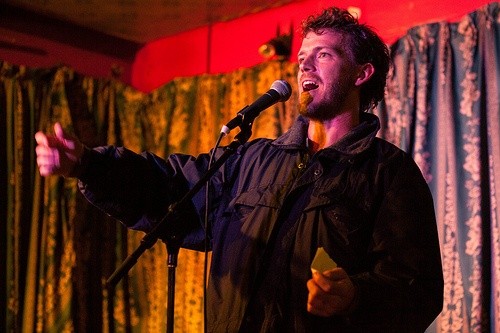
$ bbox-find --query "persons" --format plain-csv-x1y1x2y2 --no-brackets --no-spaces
35,6,445,333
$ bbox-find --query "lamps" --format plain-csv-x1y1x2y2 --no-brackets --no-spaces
259,36,292,60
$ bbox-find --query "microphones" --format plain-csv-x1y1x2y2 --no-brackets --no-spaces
220,79,293,137
